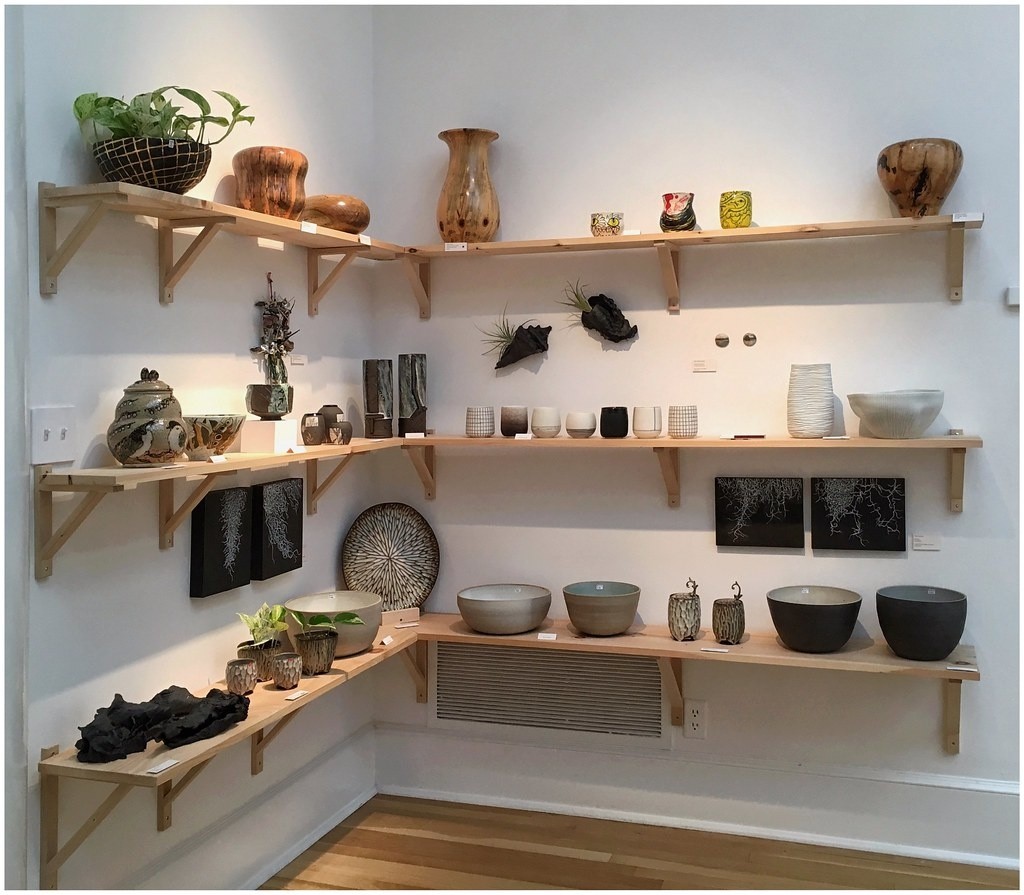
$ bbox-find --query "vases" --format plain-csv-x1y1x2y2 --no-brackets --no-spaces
270,653,301,691
226,659,256,696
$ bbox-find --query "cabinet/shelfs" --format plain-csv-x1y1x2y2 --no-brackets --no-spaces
28,179,984,890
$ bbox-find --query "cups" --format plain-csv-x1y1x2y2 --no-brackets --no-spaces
667,592,700,641
591,212,624,237
566,405,698,439
466,406,561,439
787,363,834,438
712,598,746,645
719,191,752,229
225,658,257,696
271,652,303,690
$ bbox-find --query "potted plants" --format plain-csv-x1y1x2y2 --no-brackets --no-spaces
235,603,292,681
288,608,367,674
75,86,254,195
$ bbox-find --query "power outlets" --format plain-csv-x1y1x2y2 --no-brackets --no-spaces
683,696,709,740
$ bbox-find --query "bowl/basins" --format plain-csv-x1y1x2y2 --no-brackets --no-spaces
182,414,247,461
284,591,383,657
876,585,968,661
562,581,640,635
766,585,862,654
456,584,552,635
298,194,371,234
846,389,943,439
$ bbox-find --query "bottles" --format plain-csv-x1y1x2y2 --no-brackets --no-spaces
301,404,353,446
436,128,500,243
660,192,697,232
106,367,189,467
